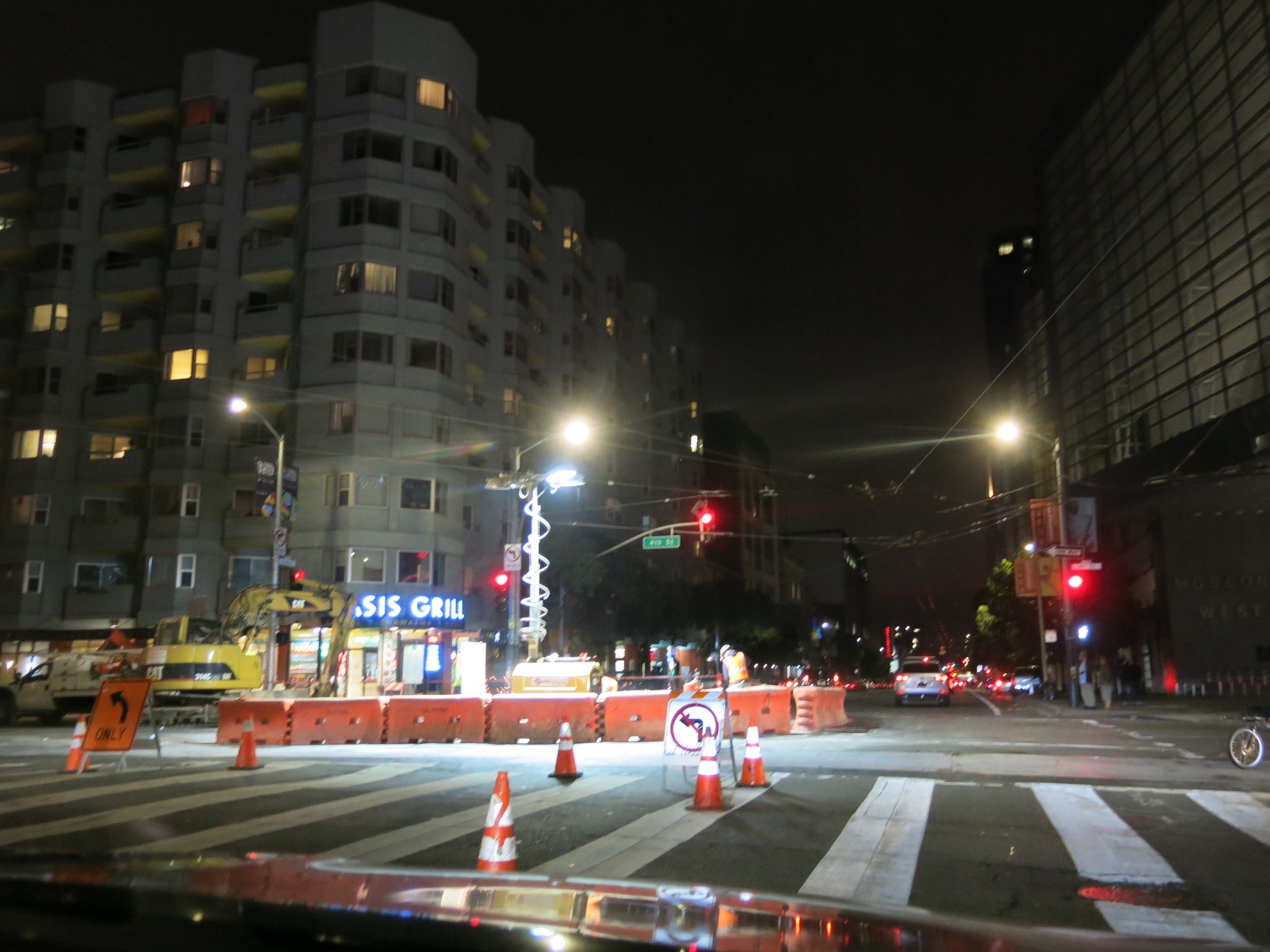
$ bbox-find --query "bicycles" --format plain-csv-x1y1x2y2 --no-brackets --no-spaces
1221,710,1270,769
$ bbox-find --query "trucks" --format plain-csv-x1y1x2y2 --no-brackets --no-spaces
3,645,143,729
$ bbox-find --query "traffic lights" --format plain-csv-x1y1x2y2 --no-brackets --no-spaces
1060,566,1091,706
699,511,713,526
493,571,509,587
286,566,306,609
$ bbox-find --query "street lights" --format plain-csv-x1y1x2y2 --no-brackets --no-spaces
1021,540,1046,689
993,420,1074,706
227,395,286,698
505,417,590,690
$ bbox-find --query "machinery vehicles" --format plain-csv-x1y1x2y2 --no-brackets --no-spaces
121,576,358,726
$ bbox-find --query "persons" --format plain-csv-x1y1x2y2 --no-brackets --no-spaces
1078,650,1098,708
720,644,751,687
1096,656,1113,708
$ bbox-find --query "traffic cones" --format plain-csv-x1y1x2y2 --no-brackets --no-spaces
224,713,267,770
684,727,734,811
734,715,771,789
546,713,584,777
476,770,520,873
56,713,98,773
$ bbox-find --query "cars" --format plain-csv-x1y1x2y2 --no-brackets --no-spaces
1010,665,1043,695
773,649,986,706
993,674,1015,694
986,676,995,693
